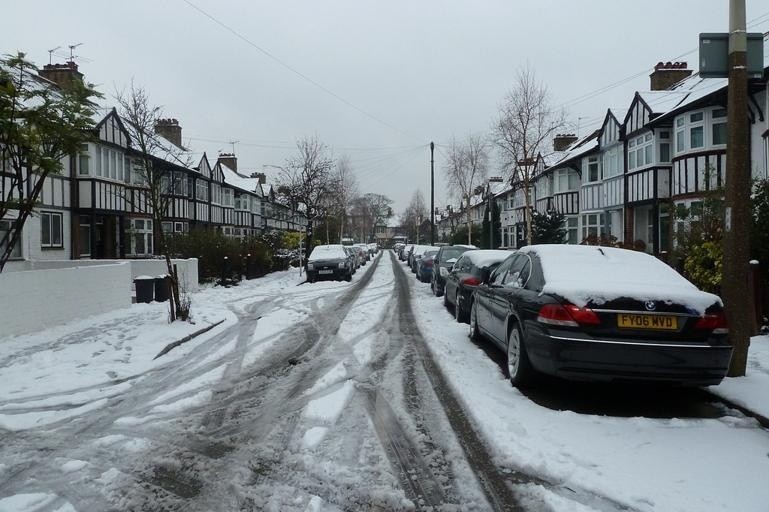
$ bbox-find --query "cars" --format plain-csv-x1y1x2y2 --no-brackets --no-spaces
394,241,516,320
304,243,379,282
471,243,734,400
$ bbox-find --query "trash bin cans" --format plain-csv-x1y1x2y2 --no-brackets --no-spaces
155,276,172,302
132,278,155,303
